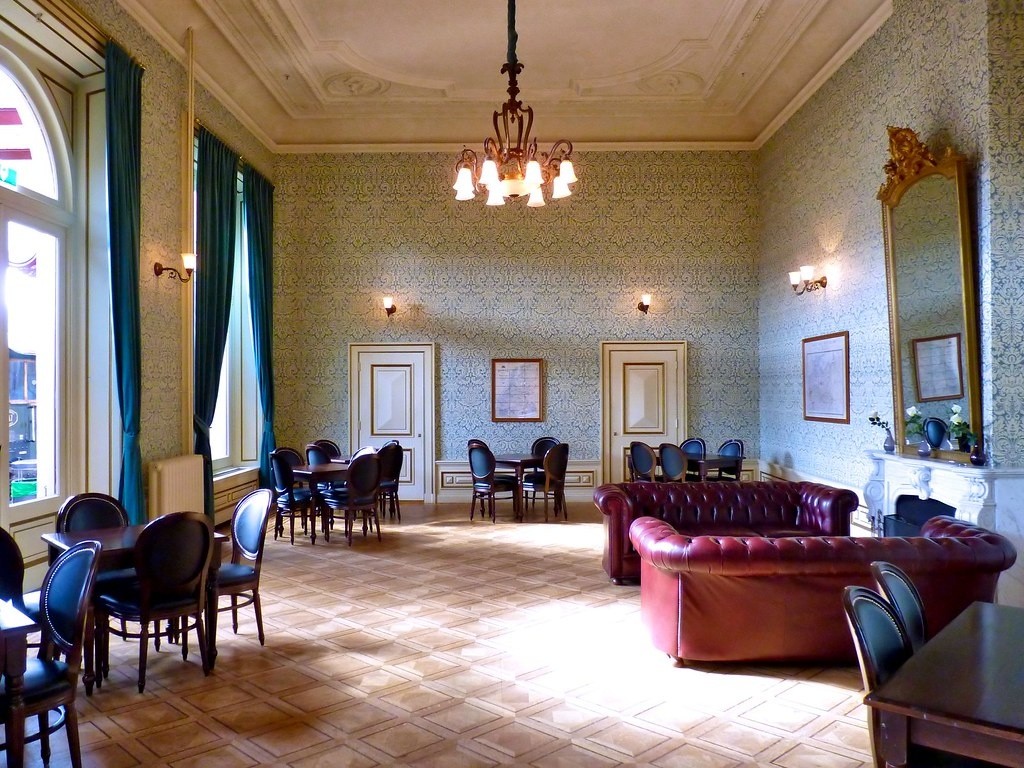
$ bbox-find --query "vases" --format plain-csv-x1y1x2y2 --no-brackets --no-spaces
884,429,894,451
956,436,973,452
915,433,931,456
967,446,986,466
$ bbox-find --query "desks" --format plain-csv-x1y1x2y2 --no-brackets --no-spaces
330,455,356,463
864,599,1024,768
626,451,746,483
291,464,348,542
492,453,544,522
0,600,39,768
37,525,230,695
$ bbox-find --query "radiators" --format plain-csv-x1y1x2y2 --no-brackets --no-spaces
148,453,203,523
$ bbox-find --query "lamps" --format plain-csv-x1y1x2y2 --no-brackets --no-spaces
638,293,652,314
789,264,827,295
153,252,197,283
453,1,577,209
383,296,396,315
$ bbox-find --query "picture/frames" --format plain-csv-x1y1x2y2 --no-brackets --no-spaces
913,332,962,403
492,358,545,423
802,331,850,424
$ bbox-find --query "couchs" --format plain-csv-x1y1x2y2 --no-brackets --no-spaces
593,480,860,585
629,516,1018,671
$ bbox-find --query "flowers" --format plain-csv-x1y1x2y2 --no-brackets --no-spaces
867,413,891,431
950,405,961,414
950,417,979,446
901,405,929,441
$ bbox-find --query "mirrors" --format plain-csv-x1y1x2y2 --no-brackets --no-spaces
879,125,982,462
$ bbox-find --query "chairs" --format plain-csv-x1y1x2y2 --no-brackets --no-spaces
0,438,404,768
842,586,914,768
869,559,922,657
658,443,690,483
717,438,745,482
628,441,657,483
678,438,706,483
465,435,568,523
922,417,949,448
